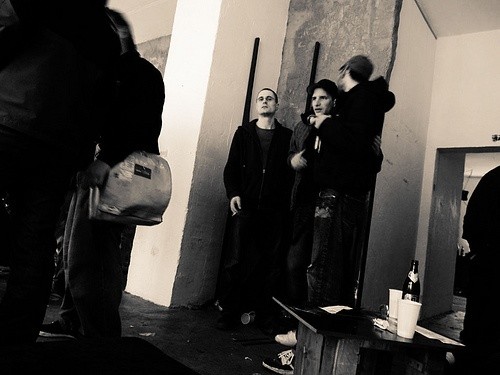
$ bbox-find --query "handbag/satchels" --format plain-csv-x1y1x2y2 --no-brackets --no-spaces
89,149,173,225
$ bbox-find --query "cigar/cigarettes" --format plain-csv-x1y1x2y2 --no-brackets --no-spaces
232,210,237,216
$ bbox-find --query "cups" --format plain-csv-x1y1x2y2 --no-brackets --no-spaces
389,289,403,319
397,299,422,339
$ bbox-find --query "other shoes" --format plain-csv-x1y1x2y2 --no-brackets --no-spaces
39,320,81,339
275,330,297,347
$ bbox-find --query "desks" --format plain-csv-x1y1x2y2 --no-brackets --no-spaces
272,295,466,375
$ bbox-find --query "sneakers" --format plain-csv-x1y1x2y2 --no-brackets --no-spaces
263,349,295,375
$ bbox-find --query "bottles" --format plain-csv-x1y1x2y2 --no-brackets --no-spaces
402,260,421,302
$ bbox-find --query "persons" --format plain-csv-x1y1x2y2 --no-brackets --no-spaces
1,0,166,375
458,166,500,375
214,89,295,331
262,56,395,375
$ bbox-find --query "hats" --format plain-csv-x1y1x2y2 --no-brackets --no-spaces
305,78,338,98
337,54,374,79
